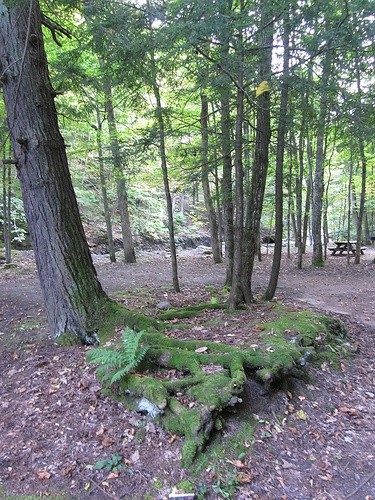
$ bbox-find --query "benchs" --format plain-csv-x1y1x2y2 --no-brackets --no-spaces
328,247,366,255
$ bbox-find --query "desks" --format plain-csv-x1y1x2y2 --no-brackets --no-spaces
331,241,357,256
